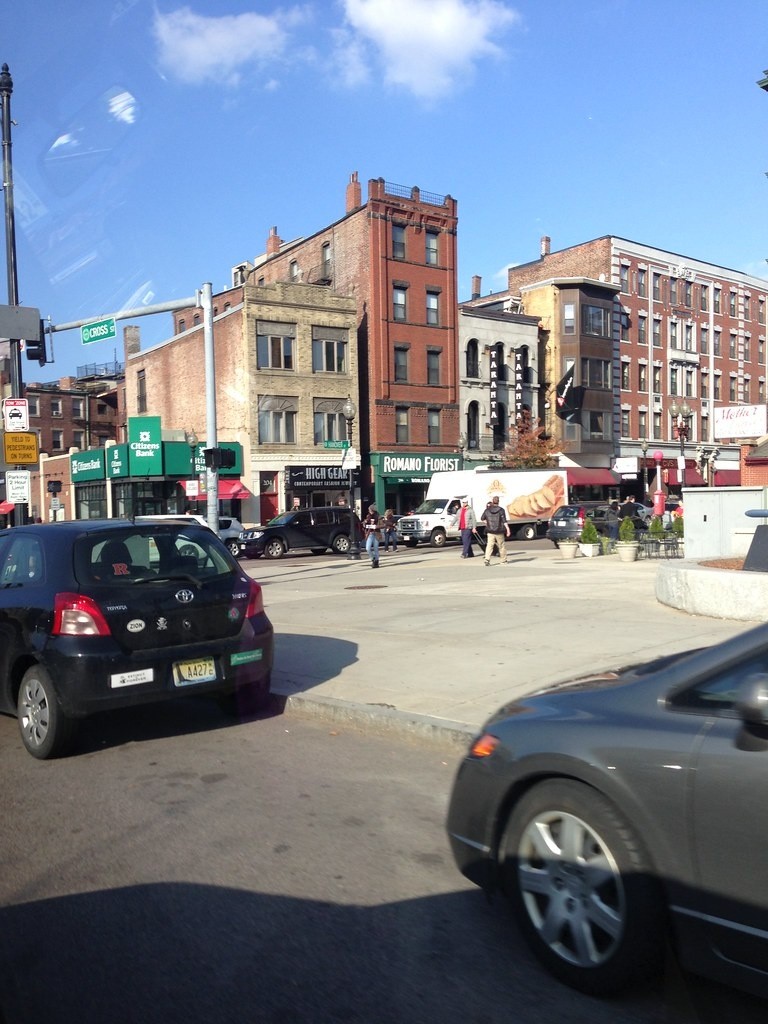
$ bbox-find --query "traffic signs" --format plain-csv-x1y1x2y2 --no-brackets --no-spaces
81,317,116,344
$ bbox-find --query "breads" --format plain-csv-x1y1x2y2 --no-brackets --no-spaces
507,475,566,518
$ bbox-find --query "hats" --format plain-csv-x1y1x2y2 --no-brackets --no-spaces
368,504,377,512
462,498,468,502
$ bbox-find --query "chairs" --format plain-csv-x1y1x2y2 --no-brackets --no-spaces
159,557,217,576
92,541,147,572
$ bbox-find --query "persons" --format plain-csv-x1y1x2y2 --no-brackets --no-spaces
383,509,399,552
450,500,477,558
675,500,684,516
604,501,618,554
617,495,640,553
405,509,414,516
363,502,385,568
481,496,511,566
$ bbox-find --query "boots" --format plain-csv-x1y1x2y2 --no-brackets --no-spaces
371,557,379,568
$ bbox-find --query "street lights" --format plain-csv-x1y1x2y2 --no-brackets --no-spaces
458,432,466,470
188,428,199,515
670,398,691,487
641,438,648,496
343,394,362,560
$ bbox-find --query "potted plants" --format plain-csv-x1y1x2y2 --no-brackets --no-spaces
640,516,683,558
558,537,578,559
615,516,639,561
578,518,600,557
600,537,614,555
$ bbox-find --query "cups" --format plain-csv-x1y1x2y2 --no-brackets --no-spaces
370,519,375,525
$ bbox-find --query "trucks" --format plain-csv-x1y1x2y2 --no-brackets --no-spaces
397,469,569,547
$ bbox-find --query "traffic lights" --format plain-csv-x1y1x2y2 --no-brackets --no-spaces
26,320,46,366
213,448,235,467
47,481,62,493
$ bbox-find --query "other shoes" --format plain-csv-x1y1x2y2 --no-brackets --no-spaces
500,560,508,565
468,554,474,557
386,550,389,552
484,559,490,566
394,550,398,552
460,555,465,559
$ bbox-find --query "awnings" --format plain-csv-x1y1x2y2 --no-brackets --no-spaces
178,480,251,500
665,469,707,486
567,467,618,486
715,469,740,486
0,500,15,514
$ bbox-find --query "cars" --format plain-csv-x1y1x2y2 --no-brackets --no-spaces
378,516,405,543
662,502,680,528
547,504,648,549
619,503,654,524
204,517,246,558
0,519,274,759
445,622,768,1000
92,515,210,563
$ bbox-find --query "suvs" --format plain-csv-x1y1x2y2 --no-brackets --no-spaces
237,506,366,560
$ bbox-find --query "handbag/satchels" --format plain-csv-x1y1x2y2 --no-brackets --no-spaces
604,510,610,526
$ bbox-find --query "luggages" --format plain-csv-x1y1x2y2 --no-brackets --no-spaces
474,531,499,557
